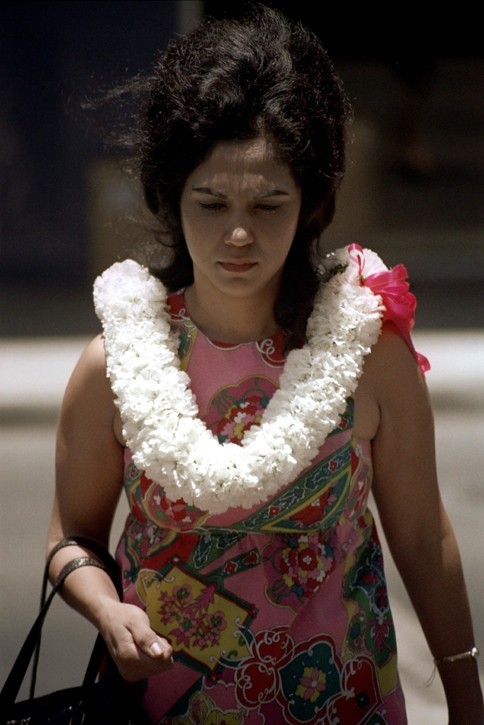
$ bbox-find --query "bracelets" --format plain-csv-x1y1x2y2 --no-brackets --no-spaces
434,647,479,666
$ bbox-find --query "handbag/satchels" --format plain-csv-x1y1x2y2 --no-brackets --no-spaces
0,537,149,725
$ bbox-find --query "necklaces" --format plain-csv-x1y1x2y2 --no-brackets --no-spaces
92,239,432,515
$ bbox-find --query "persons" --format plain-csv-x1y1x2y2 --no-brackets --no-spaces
44,4,484,725
